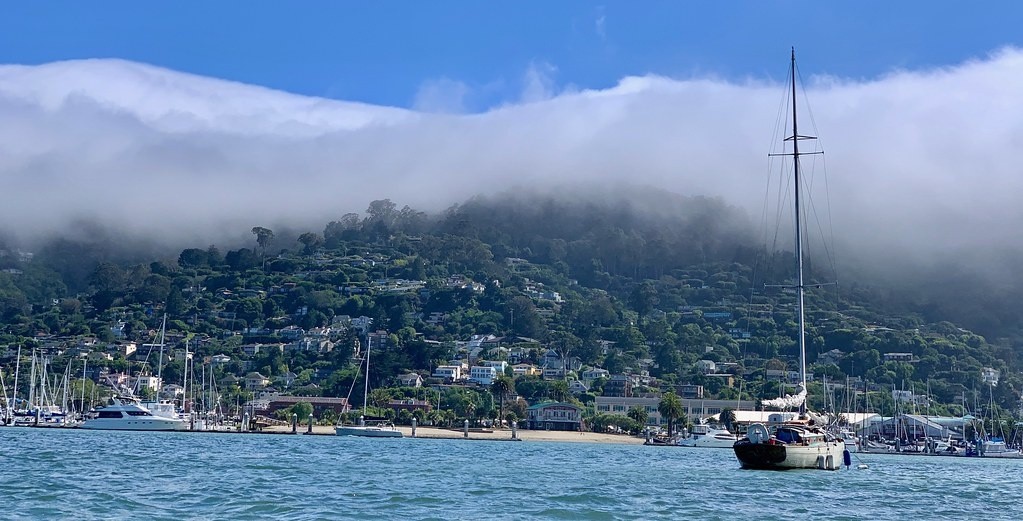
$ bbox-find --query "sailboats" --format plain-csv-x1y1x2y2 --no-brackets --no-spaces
732,44,846,470
821,375,1023,458
334,337,404,438
0,344,86,427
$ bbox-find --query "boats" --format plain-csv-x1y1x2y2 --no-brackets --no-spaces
81,397,190,430
677,425,744,448
